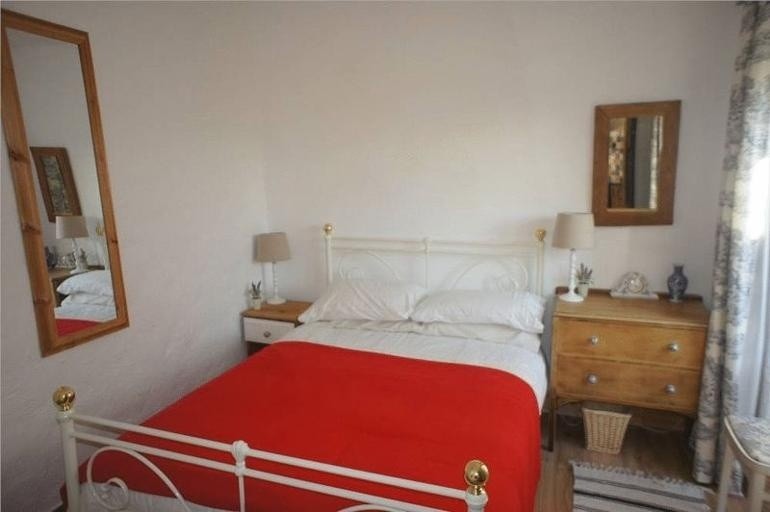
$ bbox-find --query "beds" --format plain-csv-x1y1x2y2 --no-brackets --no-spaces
54,294,117,337
53,224,551,512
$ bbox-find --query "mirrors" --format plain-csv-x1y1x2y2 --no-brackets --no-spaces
591,99,682,227
0,9,128,358
30,146,82,223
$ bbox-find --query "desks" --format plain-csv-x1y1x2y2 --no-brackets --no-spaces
548,285,711,453
48,265,104,307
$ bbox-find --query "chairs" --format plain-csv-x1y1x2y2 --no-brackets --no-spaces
716,415,770,512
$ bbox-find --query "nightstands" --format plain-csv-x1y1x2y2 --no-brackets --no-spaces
241,300,313,356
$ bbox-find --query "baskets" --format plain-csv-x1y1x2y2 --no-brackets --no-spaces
581,401,633,455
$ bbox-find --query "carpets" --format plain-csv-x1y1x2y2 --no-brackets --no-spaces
571,460,709,511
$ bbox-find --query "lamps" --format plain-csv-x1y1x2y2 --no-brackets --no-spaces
55,214,90,274
255,232,290,305
552,211,594,305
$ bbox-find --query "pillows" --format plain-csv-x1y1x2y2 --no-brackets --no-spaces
60,293,115,306
56,269,113,295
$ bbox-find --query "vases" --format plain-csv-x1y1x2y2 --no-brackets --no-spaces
668,263,688,304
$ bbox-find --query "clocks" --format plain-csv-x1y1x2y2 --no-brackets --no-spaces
628,278,643,293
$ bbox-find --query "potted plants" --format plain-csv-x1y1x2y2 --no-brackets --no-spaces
576,262,592,299
247,279,262,310
